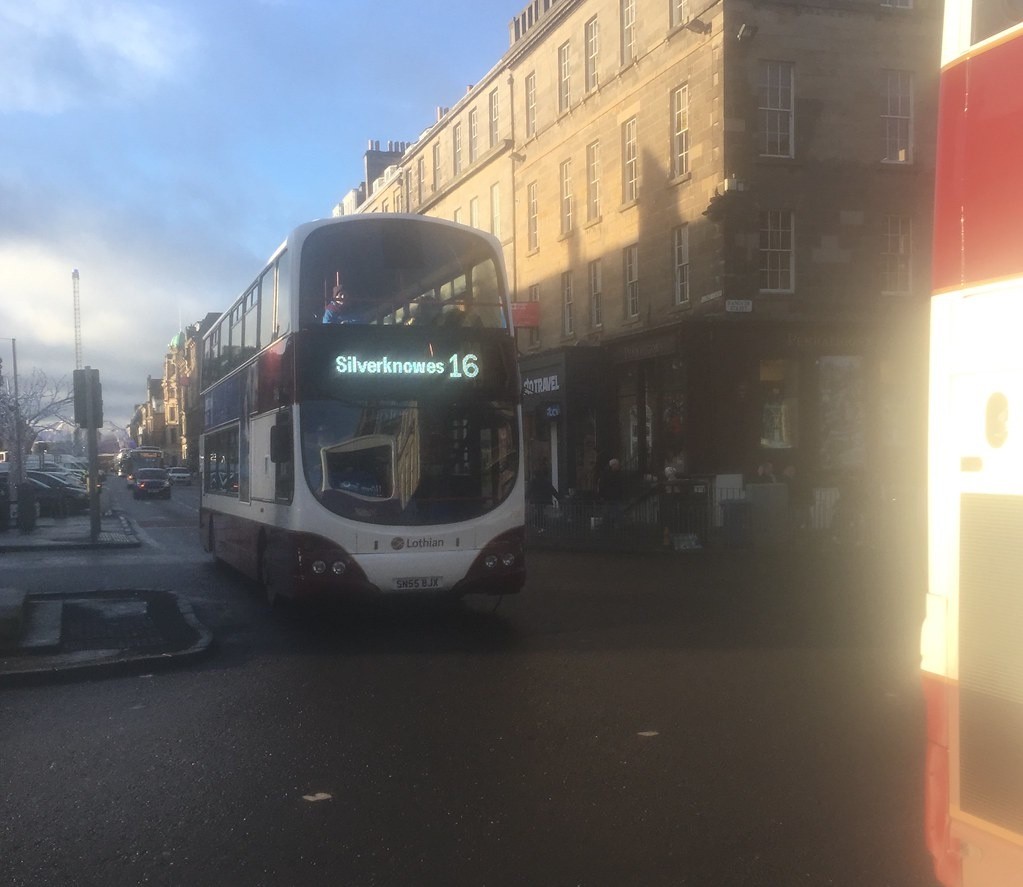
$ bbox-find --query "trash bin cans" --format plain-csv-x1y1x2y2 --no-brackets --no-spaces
664,480,713,540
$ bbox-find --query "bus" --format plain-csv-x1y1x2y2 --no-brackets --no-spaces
127,447,164,486
914,0,1022,884
185,212,530,621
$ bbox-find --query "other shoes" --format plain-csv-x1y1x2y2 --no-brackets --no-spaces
536,529,546,536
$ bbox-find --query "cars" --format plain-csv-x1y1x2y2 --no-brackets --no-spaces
135,467,173,501
168,467,191,485
0,449,132,521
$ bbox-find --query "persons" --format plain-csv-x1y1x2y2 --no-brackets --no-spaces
404,294,483,327
600,458,626,506
321,283,373,324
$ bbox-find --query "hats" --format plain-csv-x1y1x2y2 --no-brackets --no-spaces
609,458,620,466
